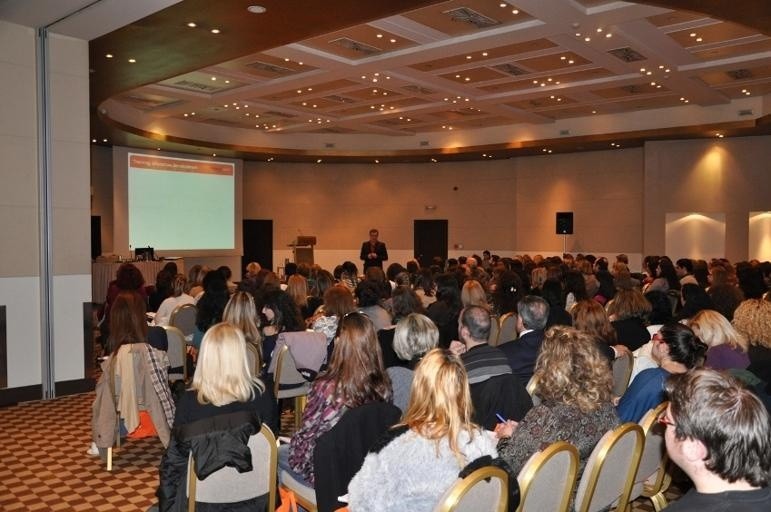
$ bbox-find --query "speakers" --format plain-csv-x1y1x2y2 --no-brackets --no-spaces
555,211,574,237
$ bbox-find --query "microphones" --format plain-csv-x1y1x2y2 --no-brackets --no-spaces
130,245,131,249
148,245,150,248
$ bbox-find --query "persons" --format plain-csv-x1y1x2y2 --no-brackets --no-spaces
361,230,388,269
88,250,771,511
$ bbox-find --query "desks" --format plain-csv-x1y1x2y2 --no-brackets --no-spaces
92,256,186,304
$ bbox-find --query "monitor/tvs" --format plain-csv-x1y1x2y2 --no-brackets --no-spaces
135,248,154,260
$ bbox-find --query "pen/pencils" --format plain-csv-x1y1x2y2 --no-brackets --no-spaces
494,412,508,425
